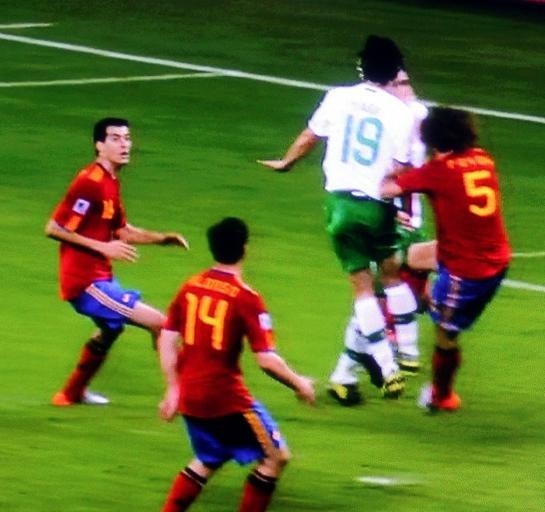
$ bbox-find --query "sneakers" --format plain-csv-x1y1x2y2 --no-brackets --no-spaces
380,376,406,400
50,391,106,407
396,359,423,376
326,377,361,406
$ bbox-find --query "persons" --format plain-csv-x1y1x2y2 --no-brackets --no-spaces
382,106,514,410
45,117,189,406
380,38,428,342
157,216,315,512
256,33,418,408
